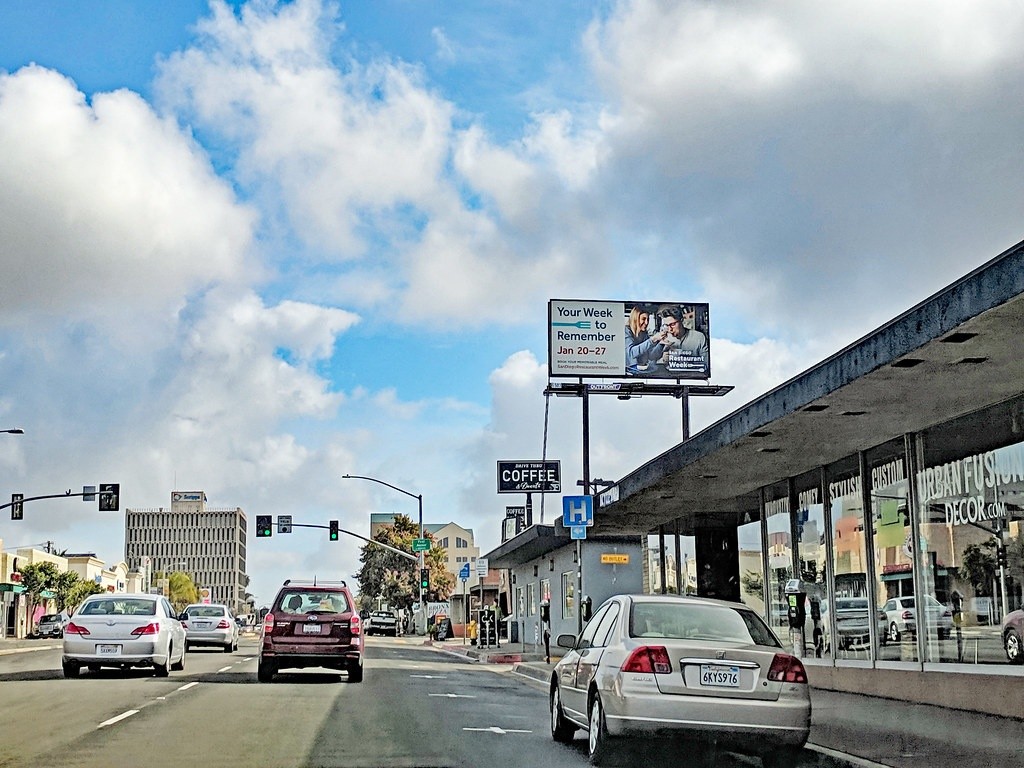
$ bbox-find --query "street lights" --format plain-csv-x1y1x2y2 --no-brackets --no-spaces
342,474,423,620
163,562,187,596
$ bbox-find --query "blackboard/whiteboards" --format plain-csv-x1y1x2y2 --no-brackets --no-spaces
437,618,455,638
478,610,499,646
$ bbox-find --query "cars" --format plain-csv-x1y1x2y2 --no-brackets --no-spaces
36,593,190,679
364,610,398,637
788,596,954,652
258,579,371,683
548,594,812,768
1002,604,1024,662
180,604,263,653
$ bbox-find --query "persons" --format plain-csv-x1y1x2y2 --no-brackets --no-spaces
625,306,709,370
490,599,504,644
288,596,302,610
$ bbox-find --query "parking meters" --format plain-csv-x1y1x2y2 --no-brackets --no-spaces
809,595,822,658
785,578,806,662
951,590,964,662
540,599,550,664
484,605,491,649
580,596,592,625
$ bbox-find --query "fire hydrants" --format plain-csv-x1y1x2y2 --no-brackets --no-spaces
467,620,479,646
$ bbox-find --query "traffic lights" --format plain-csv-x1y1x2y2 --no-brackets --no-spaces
421,569,429,588
330,521,339,541
999,546,1007,568
256,515,272,537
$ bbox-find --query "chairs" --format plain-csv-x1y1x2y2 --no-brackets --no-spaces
91,608,107,614
134,609,149,614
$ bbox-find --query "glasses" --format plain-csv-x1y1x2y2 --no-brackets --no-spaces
662,319,681,329
